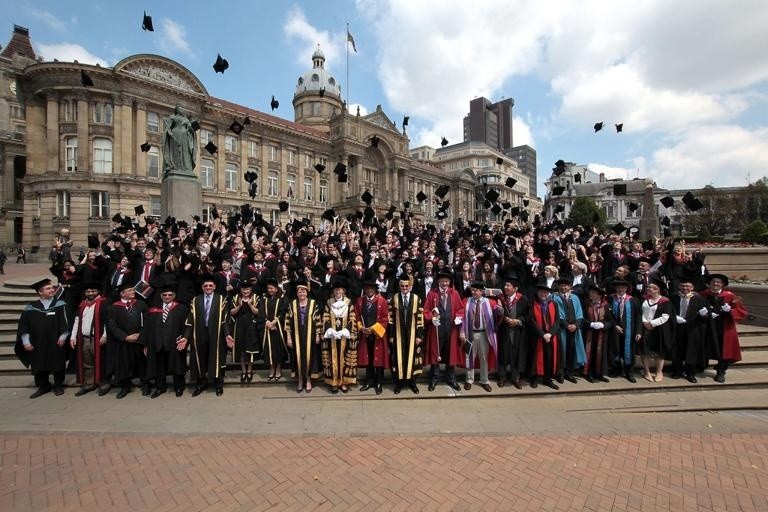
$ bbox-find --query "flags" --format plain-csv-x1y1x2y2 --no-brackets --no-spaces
348,32,357,53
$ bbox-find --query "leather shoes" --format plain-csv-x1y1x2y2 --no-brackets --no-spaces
297,382,313,393
268,372,282,380
30,385,51,398
714,374,725,382
360,380,538,395
54,385,65,396
687,375,696,383
332,386,347,394
672,371,682,379
74,383,223,398
543,371,638,390
644,373,663,382
240,372,253,381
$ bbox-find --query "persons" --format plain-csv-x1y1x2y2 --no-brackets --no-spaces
161,105,196,177
0,249,7,274
16,247,26,264
15,282,71,398
48,215,749,399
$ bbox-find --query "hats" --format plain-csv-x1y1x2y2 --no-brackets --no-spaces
87,204,728,293
594,122,603,132
371,135,379,148
213,54,229,74
505,177,517,188
435,185,449,199
552,186,565,196
362,191,373,204
244,171,258,199
334,162,348,182
523,200,529,207
141,142,150,152
416,191,427,203
314,163,325,174
81,69,95,88
682,191,704,211
496,157,503,165
229,116,250,135
552,160,566,176
613,184,627,196
484,189,500,209
143,11,154,31
271,96,279,110
205,141,217,154
31,278,51,291
574,173,581,184
615,123,622,133
660,196,674,208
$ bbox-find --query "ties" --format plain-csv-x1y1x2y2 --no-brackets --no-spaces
205,295,210,322
507,298,513,312
442,290,447,311
162,304,169,323
404,295,408,306
475,299,480,330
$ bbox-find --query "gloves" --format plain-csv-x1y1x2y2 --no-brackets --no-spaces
677,315,686,324
699,306,708,317
722,304,731,312
590,321,605,331
454,316,462,325
432,317,441,327
